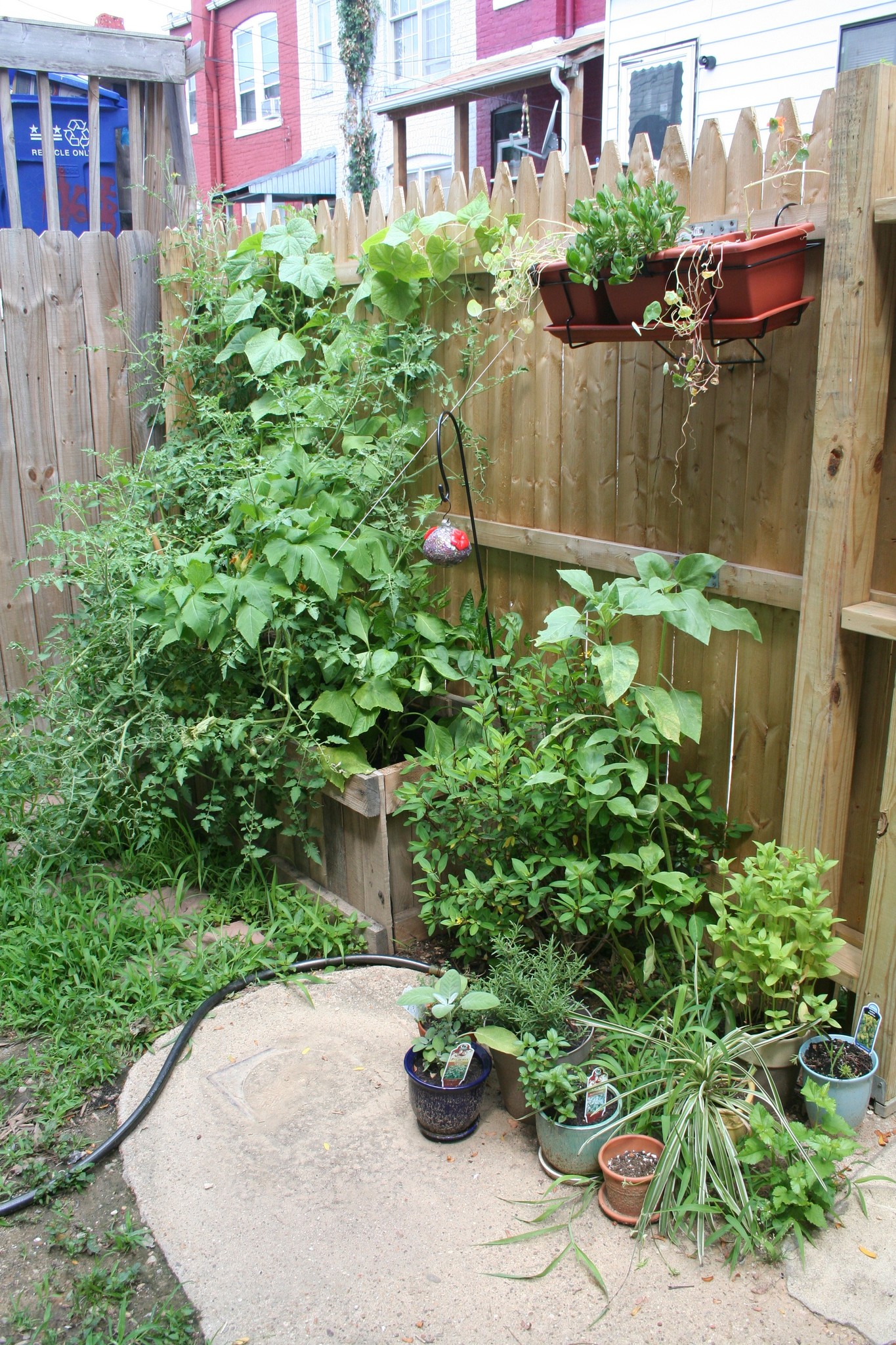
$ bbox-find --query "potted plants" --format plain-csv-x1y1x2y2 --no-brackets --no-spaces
194,585,494,953
481,918,594,1119
417,997,477,1042
512,1028,622,1176
404,1026,492,1135
789,1034,879,1138
659,1022,755,1150
704,839,848,1114
528,170,815,344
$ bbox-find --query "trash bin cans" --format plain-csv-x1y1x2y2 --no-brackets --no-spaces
0,66,129,238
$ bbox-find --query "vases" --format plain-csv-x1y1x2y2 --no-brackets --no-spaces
598,1134,665,1216
